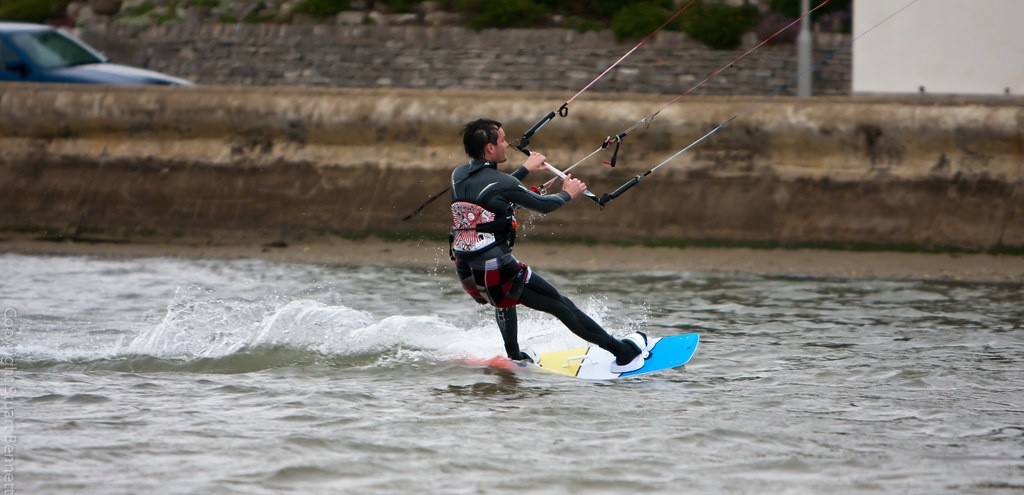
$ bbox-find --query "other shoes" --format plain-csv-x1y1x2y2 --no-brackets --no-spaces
513,347,542,364
608,329,656,373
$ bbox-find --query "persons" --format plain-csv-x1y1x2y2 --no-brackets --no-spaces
446,116,649,372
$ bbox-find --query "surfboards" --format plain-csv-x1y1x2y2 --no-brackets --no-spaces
451,331,701,381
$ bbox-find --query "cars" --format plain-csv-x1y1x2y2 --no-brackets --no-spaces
1,22,196,88
